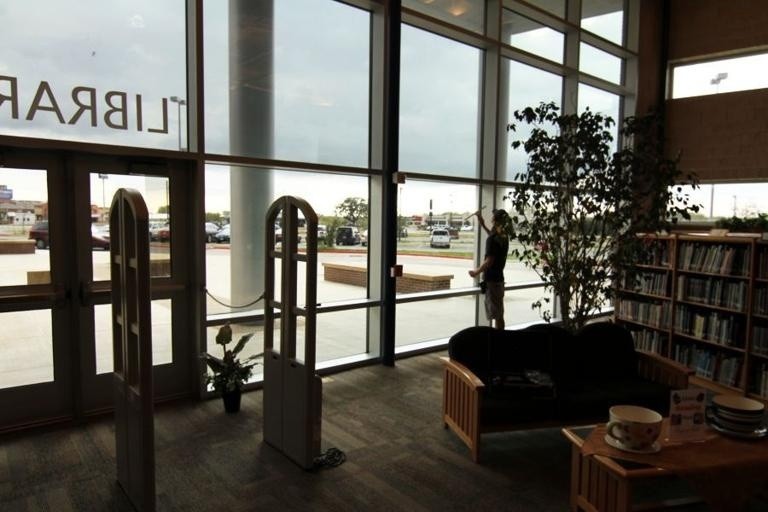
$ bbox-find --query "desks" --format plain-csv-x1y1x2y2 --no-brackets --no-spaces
560,416,768,512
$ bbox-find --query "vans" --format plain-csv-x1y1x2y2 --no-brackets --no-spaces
431,228,450,248
335,226,361,246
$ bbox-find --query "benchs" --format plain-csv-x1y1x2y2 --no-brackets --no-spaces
434,321,696,464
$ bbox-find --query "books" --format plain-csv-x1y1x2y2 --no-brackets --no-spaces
615,238,768,398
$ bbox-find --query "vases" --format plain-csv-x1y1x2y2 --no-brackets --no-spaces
222,391,242,414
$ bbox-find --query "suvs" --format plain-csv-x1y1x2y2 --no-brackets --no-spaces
29,219,51,249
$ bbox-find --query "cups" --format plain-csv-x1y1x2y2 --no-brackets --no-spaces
606,405,663,449
710,395,765,433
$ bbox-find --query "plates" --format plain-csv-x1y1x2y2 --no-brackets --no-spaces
706,422,767,439
603,435,662,455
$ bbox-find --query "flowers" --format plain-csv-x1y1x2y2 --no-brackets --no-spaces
196,319,264,392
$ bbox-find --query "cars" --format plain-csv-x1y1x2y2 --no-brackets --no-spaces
426,224,473,232
148,219,170,243
399,229,408,236
274,219,330,245
361,229,368,246
91,222,112,250
205,221,231,245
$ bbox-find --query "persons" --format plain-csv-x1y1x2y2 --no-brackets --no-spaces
469,207,515,330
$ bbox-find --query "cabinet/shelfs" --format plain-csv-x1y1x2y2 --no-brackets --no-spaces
613,235,768,409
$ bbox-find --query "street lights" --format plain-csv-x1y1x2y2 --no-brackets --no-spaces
170,94,187,151
398,187,403,242
710,71,729,220
98,175,108,218
